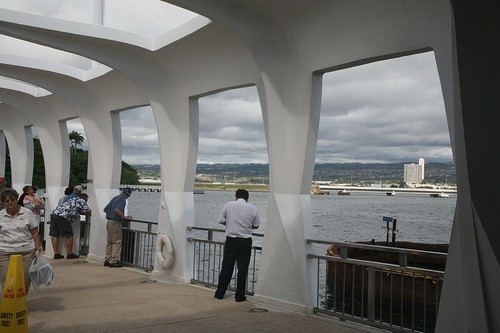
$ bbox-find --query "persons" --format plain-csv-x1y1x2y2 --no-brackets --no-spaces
104,187,132,267
0,177,91,297
214,189,260,302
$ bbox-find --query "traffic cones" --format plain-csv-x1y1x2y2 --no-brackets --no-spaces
0,255,30,333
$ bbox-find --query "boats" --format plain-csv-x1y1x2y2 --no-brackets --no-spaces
325,216,450,306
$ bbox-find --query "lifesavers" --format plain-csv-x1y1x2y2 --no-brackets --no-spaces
155,233,176,270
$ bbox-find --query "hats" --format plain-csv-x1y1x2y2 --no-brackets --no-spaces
74,185,82,191
123,187,133,196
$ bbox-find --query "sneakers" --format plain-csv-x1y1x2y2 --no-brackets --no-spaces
104,262,111,266
109,262,122,266
66,254,79,259
54,254,64,259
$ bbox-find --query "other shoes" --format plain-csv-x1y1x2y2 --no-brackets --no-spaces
214,293,223,298
236,297,246,303
